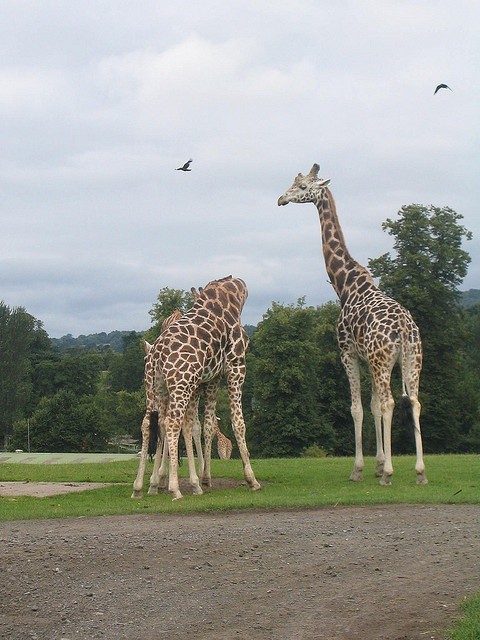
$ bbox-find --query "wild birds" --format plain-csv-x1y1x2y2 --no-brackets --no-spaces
174,160,193,172
435,82,453,95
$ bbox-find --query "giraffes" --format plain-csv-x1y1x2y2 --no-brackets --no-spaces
214,416,232,464
276,162,430,489
130,276,261,500
